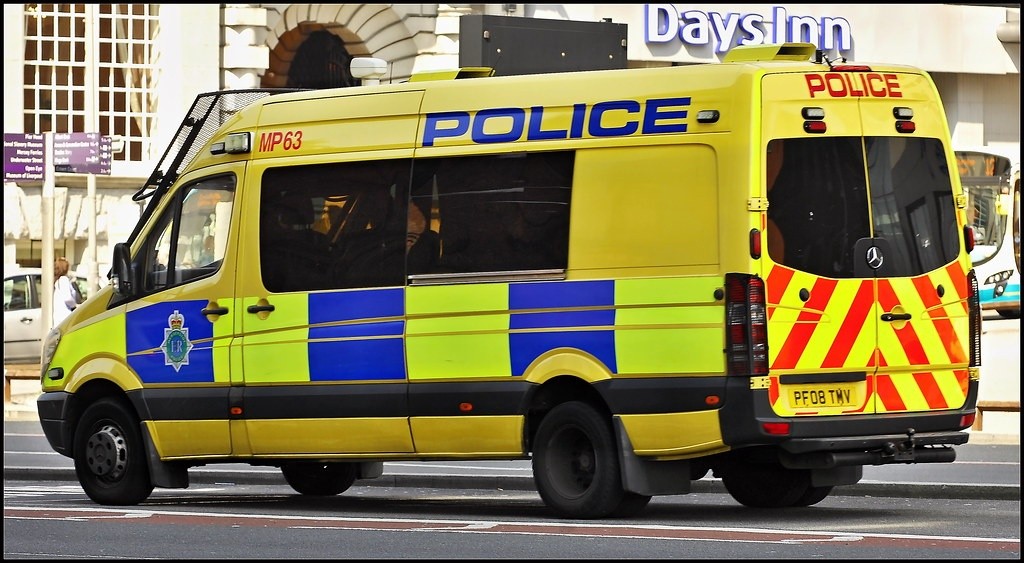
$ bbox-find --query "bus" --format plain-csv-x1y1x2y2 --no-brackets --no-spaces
951,143,1020,318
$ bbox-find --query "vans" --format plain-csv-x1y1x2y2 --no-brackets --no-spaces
36,42,984,519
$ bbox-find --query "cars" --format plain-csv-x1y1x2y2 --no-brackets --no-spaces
3,268,104,361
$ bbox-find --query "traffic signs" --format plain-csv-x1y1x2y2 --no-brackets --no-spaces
52,133,100,165
3,134,44,180
56,138,112,176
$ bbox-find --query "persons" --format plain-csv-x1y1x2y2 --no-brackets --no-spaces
201,184,282,267
53,257,80,327
